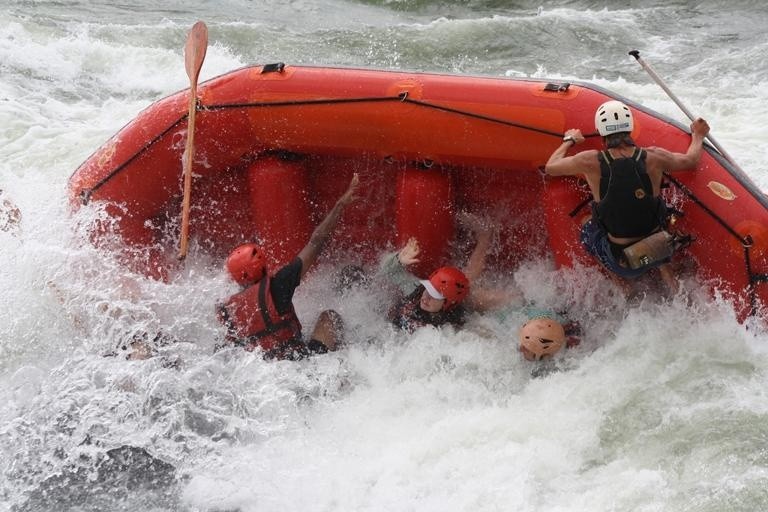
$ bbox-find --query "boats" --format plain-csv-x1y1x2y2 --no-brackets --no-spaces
63,61,768,334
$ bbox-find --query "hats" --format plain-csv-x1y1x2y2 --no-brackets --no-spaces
419,279,444,300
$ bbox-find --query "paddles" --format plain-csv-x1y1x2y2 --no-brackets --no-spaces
178,22,208,260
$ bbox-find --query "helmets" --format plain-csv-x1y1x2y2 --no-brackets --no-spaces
595,101,635,137
521,319,565,357
430,267,468,310
228,244,267,286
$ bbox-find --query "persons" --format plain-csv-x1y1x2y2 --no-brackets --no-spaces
213,172,362,361
379,227,468,330
544,101,711,305
454,209,585,373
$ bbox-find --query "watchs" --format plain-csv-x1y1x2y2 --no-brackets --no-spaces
562,135,576,147
